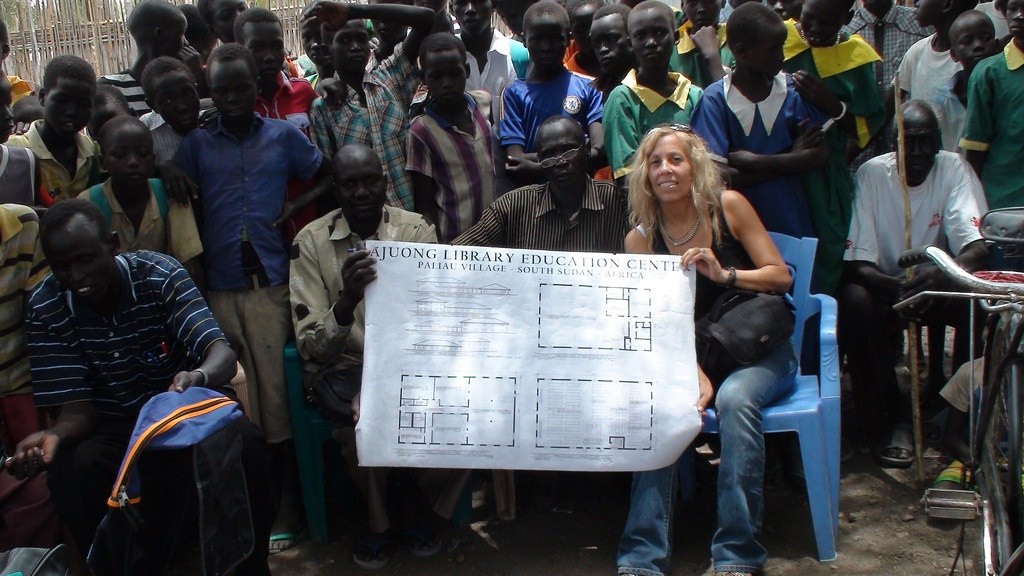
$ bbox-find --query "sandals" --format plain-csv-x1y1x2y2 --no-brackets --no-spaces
353,528,394,569
878,422,915,465
401,519,441,556
924,412,947,443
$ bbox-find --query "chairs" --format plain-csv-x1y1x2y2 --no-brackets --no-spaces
701,230,851,562
284,342,474,545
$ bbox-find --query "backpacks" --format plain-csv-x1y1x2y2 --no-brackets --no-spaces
85,387,273,576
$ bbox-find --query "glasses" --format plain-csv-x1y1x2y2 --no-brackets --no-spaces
538,140,585,168
646,123,694,135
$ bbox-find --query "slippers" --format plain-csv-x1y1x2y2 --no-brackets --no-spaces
268,525,308,554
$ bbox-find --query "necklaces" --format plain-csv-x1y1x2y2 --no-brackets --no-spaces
801,28,841,48
658,211,700,246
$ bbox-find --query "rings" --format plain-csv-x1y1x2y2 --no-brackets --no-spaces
699,248,704,253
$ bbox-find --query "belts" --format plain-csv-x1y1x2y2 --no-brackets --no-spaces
232,272,270,291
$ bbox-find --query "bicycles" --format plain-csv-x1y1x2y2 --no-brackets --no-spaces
892,207,1024,576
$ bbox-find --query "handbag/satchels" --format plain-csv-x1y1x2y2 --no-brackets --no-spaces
0,544,71,576
694,289,796,406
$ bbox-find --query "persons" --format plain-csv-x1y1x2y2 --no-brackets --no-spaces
5,198,283,576
289,143,443,569
842,99,996,466
0,449,92,575
447,116,625,504
0,0,1024,553
617,123,800,576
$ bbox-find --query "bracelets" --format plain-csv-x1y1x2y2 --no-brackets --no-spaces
721,266,736,290
191,368,209,387
